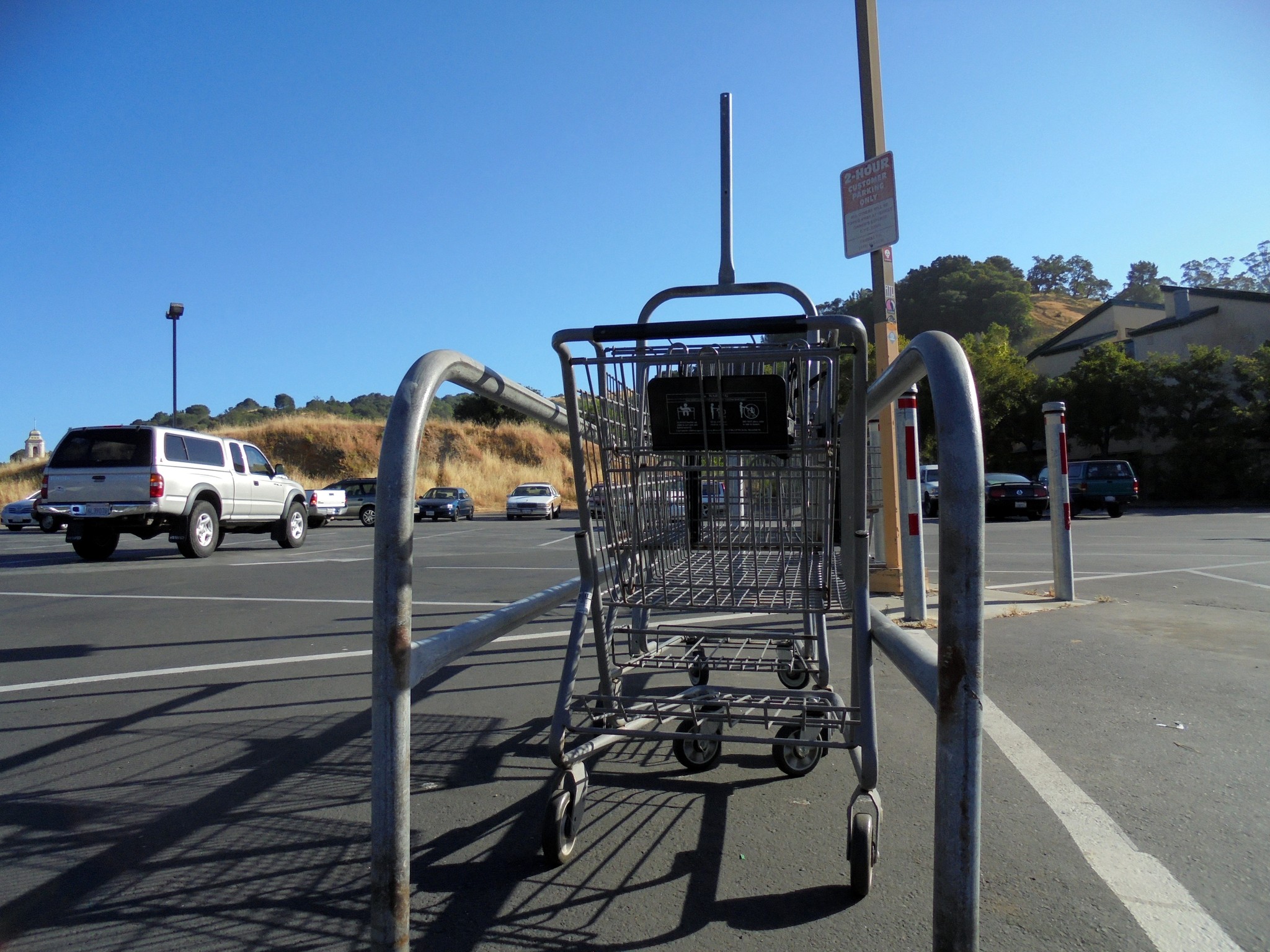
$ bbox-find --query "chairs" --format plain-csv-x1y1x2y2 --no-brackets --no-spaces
522,491,529,494
370,488,376,494
347,491,353,495
356,489,362,495
540,490,546,495
453,493,457,496
435,493,441,498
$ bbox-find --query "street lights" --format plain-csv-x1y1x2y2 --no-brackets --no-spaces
165,303,185,430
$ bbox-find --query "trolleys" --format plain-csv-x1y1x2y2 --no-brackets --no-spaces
540,305,884,899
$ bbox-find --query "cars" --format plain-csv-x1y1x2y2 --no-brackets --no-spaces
412,487,475,523
919,464,939,518
319,477,420,528
984,472,1050,523
0,489,68,532
588,474,728,531
505,482,561,521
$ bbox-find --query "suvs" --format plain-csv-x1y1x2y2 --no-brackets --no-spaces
1037,459,1139,519
31,423,309,560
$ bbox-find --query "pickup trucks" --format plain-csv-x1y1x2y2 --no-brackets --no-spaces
304,489,349,528
